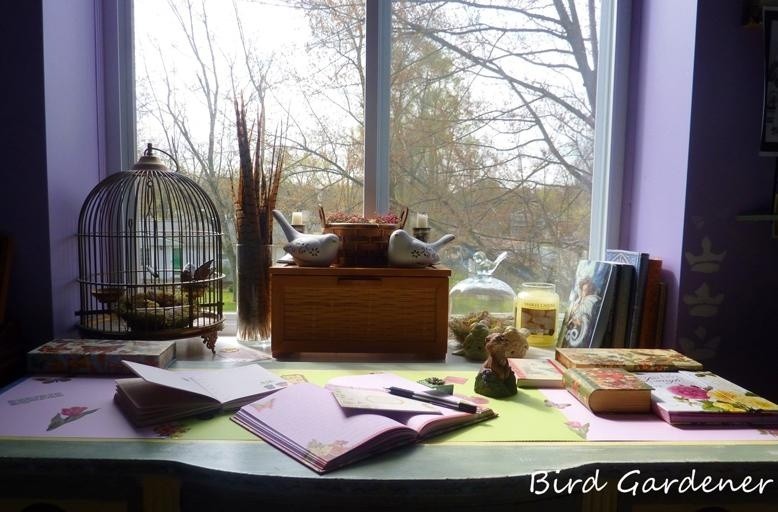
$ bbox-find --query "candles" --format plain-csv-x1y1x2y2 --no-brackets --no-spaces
290,210,303,225
415,212,428,229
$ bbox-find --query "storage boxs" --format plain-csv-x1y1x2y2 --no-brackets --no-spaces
262,250,454,365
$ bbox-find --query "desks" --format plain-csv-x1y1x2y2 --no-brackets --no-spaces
0,300,777,512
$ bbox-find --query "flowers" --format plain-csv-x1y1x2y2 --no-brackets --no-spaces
322,206,402,226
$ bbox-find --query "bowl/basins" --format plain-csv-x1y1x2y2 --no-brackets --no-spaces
91,287,124,303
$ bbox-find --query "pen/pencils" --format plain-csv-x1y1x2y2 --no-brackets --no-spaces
384,385,477,415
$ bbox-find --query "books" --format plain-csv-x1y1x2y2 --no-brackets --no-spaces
507,347,777,428
232,369,496,477
559,247,669,350
112,360,290,428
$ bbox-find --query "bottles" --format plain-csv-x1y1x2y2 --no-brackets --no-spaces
512,281,559,347
